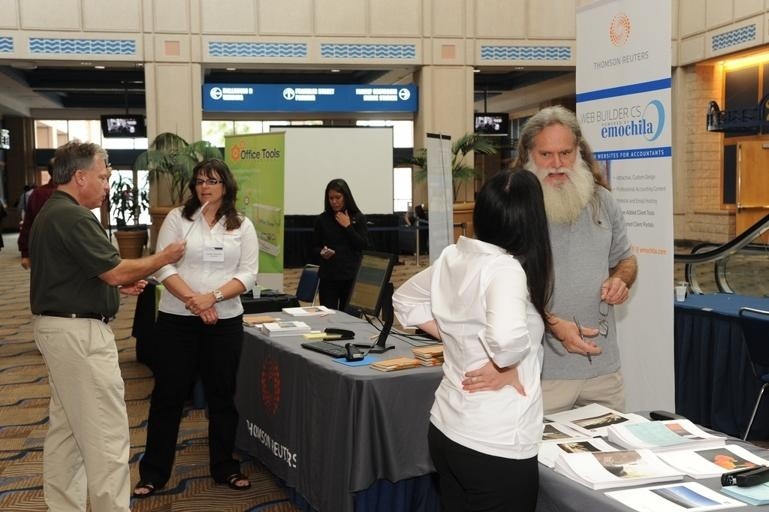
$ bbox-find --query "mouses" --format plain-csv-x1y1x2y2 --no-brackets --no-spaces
346,353,364,362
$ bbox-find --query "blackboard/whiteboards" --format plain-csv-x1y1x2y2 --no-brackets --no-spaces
268,126,395,219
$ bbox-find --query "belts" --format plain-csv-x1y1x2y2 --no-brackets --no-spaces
39,310,110,324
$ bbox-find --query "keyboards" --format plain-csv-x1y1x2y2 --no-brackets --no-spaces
301,340,364,358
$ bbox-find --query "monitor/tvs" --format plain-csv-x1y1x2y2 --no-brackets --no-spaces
474,112,509,136
101,114,146,137
344,249,399,354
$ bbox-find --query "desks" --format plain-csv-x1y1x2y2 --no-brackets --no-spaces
537,407,769,511
145,281,298,408
233,307,443,511
675,291,768,438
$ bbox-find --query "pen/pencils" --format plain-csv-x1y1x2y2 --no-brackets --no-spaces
574,314,595,367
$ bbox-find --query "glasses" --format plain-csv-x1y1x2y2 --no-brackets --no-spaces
595,286,608,339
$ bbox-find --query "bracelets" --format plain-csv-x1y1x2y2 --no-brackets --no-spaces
212,288,227,304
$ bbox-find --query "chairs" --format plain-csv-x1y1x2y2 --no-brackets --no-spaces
737,307,768,440
296,263,320,305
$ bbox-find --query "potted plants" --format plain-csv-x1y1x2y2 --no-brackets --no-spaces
106,175,152,260
148,130,226,239
406,129,500,244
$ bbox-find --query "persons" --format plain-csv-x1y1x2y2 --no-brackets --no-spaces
28,139,187,512
512,102,640,418
133,155,262,500
313,178,371,310
391,166,557,511
14,180,40,253
0,198,9,256
17,157,59,271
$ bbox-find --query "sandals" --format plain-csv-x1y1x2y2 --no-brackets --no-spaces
132,480,156,498
226,471,251,490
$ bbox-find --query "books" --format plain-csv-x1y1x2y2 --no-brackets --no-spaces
539,398,769,511
241,302,446,373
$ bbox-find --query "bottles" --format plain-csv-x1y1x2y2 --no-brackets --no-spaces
707,100,720,130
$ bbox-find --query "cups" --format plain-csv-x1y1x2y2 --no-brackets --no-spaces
251,285,261,298
674,281,689,302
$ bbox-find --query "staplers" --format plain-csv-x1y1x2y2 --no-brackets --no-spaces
323,328,355,341
721,466,769,487
